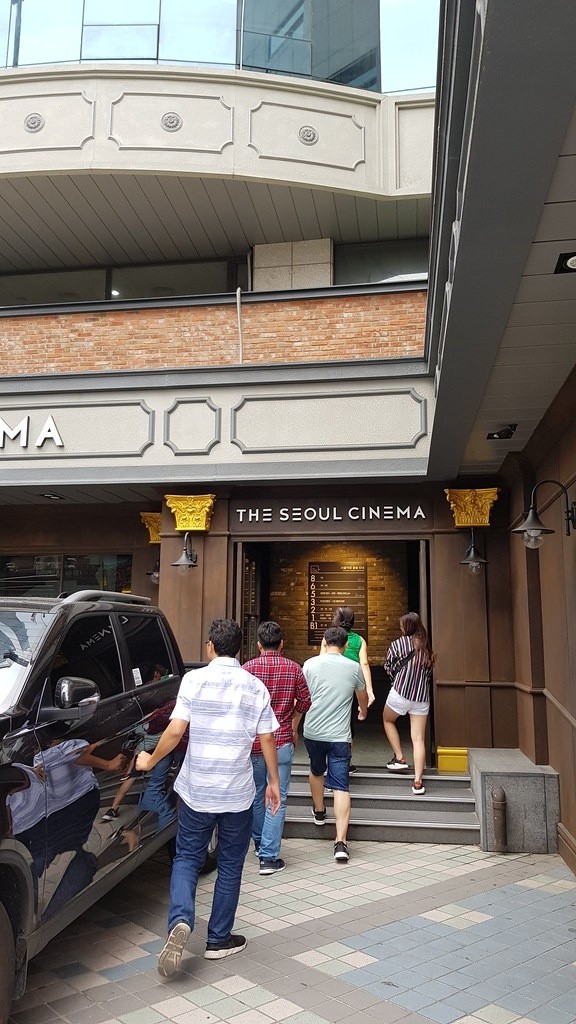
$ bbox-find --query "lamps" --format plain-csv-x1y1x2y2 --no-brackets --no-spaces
459,525,490,576
146,549,160,586
511,480,576,549
170,530,199,570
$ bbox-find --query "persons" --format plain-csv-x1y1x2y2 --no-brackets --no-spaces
4,700,190,886
384,612,435,794
241,622,312,874
319,607,375,773
302,627,368,861
135,619,281,976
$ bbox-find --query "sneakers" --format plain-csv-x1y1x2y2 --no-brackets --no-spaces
312,805,327,825
157,919,191,978
411,782,425,794
204,934,247,959
348,765,358,773
386,753,408,770
260,859,286,874
111,825,125,839
102,807,120,821
255,841,261,857
333,841,349,860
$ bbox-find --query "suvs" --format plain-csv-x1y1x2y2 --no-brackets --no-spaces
0,587,218,1024
